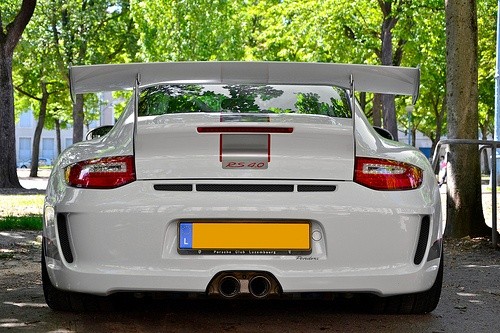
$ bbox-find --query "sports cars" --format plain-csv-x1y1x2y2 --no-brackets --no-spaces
39,60,446,316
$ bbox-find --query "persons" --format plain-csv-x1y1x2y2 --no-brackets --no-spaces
437,156,446,185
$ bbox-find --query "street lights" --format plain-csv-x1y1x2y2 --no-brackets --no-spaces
405,106,414,146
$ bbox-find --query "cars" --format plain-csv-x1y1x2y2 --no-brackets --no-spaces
20,157,51,169
